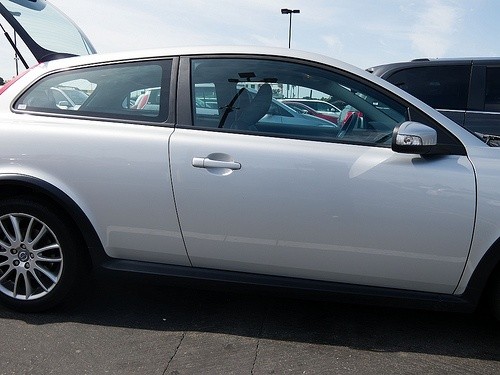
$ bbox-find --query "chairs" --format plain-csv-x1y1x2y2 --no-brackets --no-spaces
227,84,272,131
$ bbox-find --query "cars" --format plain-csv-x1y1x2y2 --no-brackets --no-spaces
1,0,500,142
0,42,500,333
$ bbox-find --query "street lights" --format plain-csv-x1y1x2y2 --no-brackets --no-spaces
281,9,300,98
